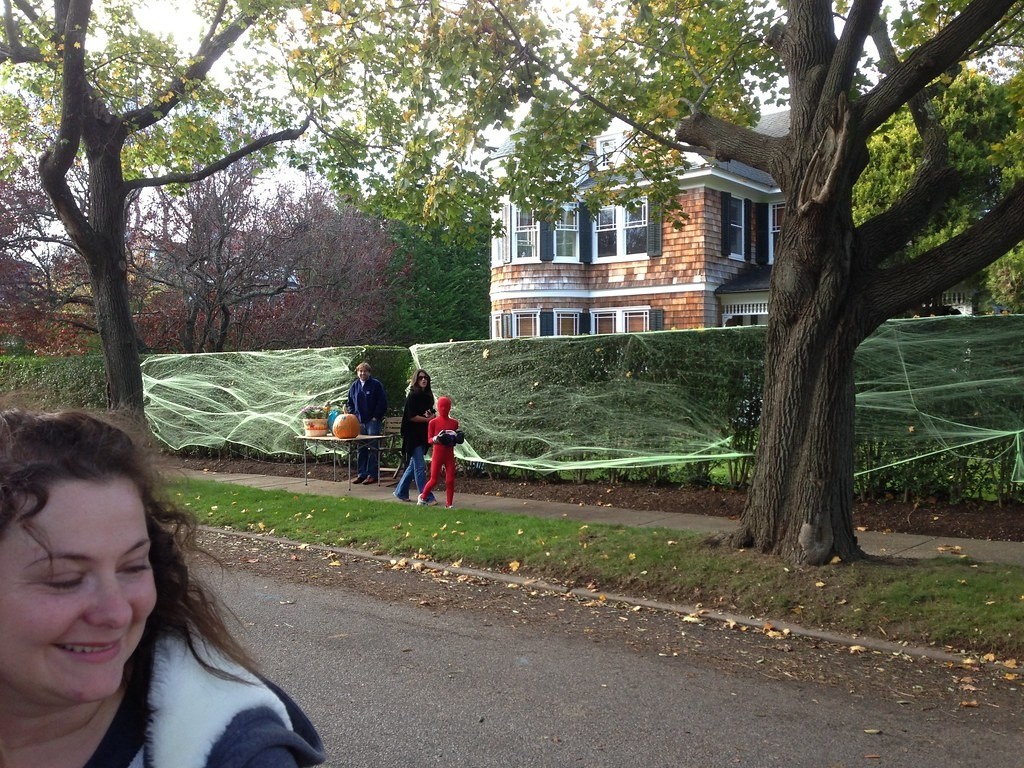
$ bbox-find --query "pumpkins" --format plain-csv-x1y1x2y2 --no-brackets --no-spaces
333,404,360,439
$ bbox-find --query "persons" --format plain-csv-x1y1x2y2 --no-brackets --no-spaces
392,369,465,509
348,363,387,485
0,409,326,768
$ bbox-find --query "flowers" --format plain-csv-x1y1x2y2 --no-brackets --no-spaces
302,406,330,419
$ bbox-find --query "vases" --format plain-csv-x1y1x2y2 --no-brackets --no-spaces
303,419,328,437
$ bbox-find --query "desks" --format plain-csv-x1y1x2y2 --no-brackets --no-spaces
294,432,387,491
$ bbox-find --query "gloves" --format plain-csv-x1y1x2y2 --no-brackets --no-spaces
433,430,449,445
455,429,464,444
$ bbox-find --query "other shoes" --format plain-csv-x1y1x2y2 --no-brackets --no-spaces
445,505,453,509
394,491,411,502
417,492,426,507
362,475,375,484
353,477,364,484
420,497,437,507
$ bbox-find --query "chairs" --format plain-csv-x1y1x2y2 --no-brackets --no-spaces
380,417,408,478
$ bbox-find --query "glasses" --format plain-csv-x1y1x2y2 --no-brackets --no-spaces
418,375,430,380
